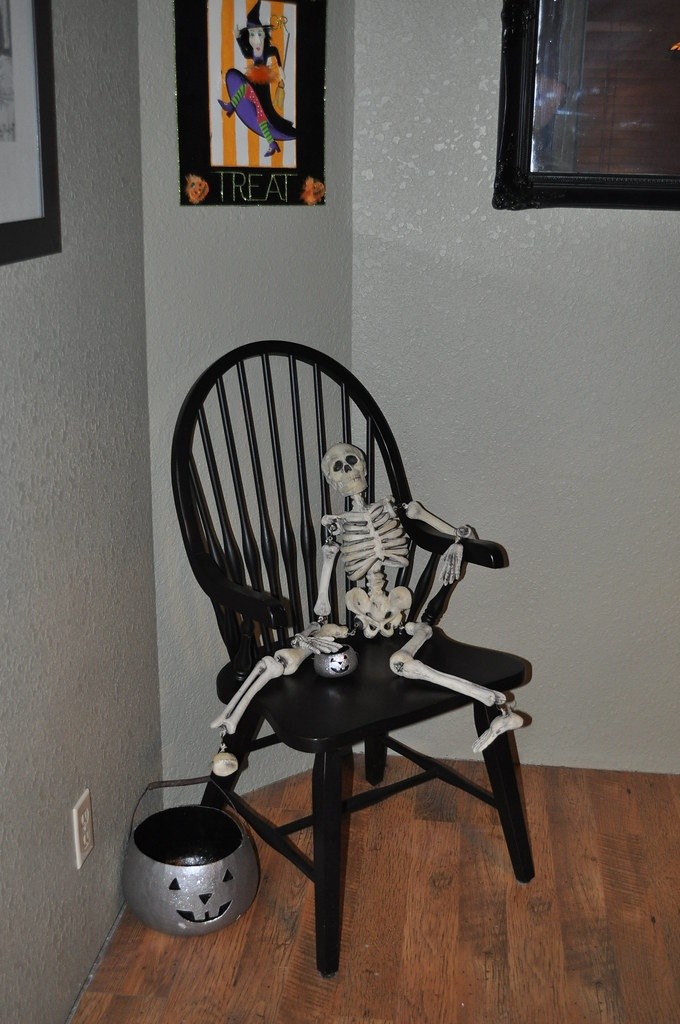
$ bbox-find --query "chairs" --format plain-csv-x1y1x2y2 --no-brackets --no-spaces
172,340,538,980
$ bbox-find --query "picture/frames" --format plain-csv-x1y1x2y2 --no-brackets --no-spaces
174,0,326,207
0,0,63,267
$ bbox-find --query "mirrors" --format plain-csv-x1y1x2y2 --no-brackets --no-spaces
491,0,680,211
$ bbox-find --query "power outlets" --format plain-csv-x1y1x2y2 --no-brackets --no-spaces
72,789,95,868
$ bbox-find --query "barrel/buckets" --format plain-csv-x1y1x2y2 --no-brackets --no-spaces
122,777,261,937
